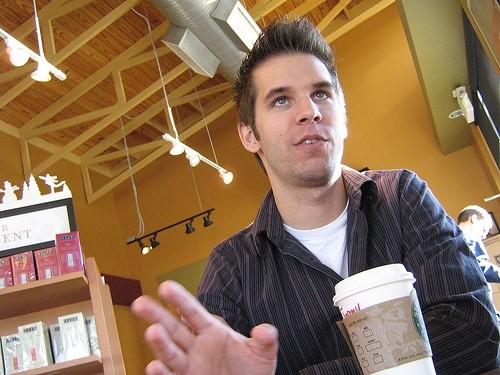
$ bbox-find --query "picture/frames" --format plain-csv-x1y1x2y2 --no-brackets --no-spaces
0,198,77,257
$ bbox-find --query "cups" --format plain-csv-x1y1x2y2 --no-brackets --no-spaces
332,263,437,375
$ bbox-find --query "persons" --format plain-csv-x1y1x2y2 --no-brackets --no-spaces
457,205,500,283
131,13,500,375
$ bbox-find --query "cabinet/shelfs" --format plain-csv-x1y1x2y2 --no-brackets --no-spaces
0,258,127,375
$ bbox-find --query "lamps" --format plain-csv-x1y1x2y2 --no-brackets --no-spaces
120,100,215,256
142,0,233,185
449,85,474,123
0,0,67,83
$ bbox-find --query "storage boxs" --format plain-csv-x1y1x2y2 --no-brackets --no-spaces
0,231,85,288
1,311,102,375
